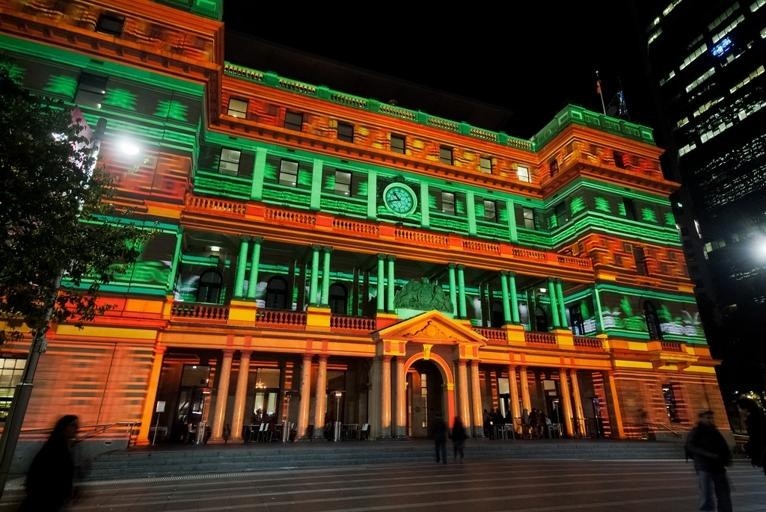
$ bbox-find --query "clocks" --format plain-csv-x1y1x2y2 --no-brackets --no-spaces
383,182,418,218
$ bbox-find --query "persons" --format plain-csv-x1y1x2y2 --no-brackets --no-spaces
26,414,93,512
736,398,766,475
682,410,734,511
428,406,554,464
393,275,450,311
248,405,335,441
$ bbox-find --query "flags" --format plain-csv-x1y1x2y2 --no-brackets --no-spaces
592,75,601,94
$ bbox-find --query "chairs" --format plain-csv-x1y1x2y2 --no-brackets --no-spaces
188,422,197,445
355,424,370,441
244,423,281,447
495,423,515,440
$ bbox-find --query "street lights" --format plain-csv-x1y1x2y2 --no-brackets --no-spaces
1,118,140,502
